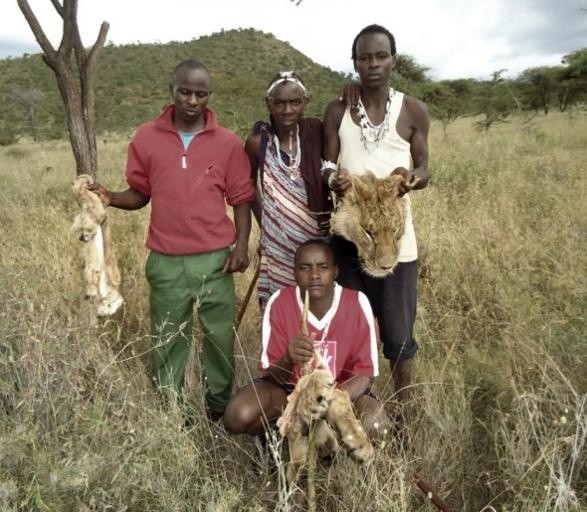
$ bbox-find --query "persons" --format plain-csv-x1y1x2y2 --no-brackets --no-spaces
323,23,430,424
84,60,256,430
243,72,361,314
224,240,389,478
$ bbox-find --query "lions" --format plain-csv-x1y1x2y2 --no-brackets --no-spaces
329,162,425,277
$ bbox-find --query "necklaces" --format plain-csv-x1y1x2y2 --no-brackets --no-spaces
273,122,301,174
356,86,393,154
